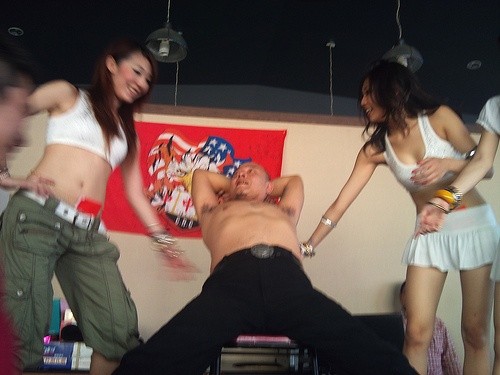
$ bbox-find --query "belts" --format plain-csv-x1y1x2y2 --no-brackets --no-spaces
21,188,107,233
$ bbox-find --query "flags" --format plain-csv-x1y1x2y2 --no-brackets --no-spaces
99,118,289,238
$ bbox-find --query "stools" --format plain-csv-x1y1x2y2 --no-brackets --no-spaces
210,335,319,375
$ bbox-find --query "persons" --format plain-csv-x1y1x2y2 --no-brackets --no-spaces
108,160,419,375
0,61,30,157
412,97,500,374
0,41,205,375
398,280,462,375
297,60,499,375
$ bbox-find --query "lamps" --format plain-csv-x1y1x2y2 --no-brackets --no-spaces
143,0,188,62
381,0,424,74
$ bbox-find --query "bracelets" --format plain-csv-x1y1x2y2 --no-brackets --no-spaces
296,241,316,258
427,185,463,214
319,216,338,229
0,165,10,182
145,221,185,261
